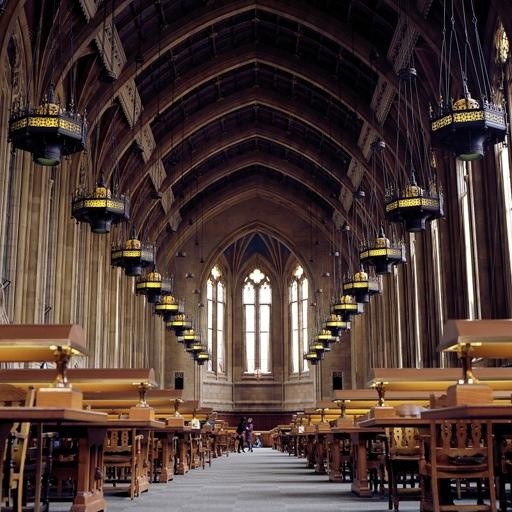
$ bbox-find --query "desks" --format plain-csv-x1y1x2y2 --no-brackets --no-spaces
1,420,167,501
82,390,221,482
1,406,108,512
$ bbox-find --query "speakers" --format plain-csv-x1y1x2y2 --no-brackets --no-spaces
175,372,183,389
333,371,342,390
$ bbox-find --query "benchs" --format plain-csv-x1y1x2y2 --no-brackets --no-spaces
287,366,512,511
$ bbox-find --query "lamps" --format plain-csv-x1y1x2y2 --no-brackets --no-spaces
193,1,210,366
10,0,90,167
329,3,364,322
342,2,379,303
308,2,332,360
383,3,444,233
1,367,156,420
314,3,340,348
152,1,184,323
359,3,406,276
186,0,207,359
110,1,158,277
134,1,174,305
70,1,130,235
430,1,507,163
1,324,90,407
165,1,193,337
177,1,201,349
302,0,324,367
321,2,351,337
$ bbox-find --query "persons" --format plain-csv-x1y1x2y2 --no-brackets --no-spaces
252,436,262,448
244,418,254,453
235,416,249,453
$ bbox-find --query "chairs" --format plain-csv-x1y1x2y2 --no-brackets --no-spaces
2,383,35,512
